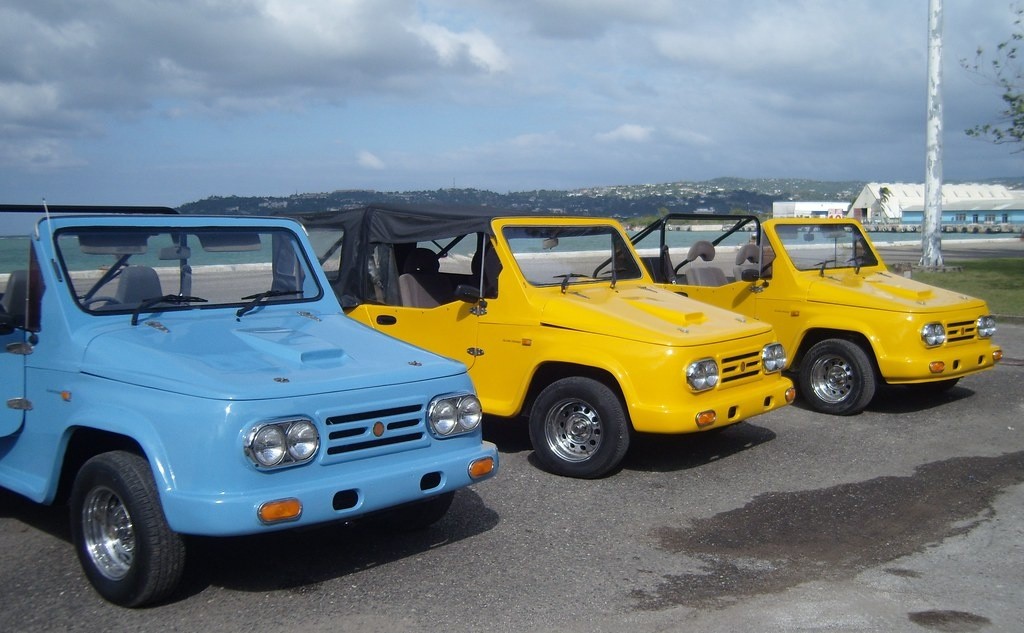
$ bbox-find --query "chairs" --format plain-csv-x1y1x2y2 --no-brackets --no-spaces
0,270,28,315
453,241,502,295
115,266,162,304
684,241,728,287
399,248,451,308
732,243,770,281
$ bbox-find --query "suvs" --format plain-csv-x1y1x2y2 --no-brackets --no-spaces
281,202,794,477
577,213,1005,417
0,205,498,610
